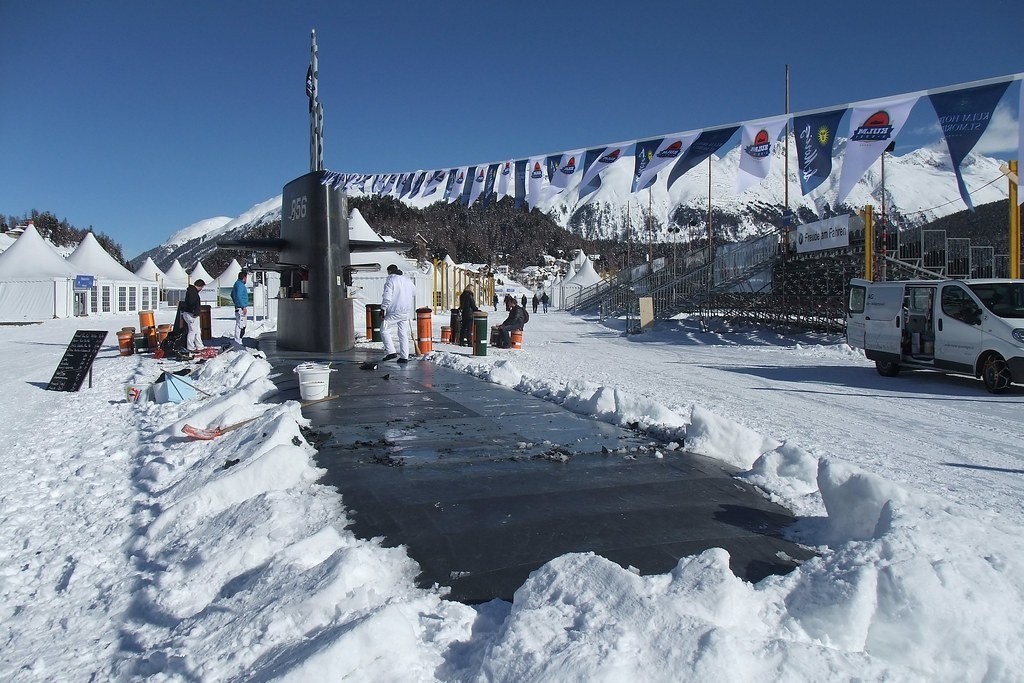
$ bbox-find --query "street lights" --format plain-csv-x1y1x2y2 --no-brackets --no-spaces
881,142,904,281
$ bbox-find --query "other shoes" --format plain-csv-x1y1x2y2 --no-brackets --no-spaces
498,345,510,348
383,353,397,361
460,343,465,346
359,362,378,370
468,343,473,346
495,344,502,347
397,358,408,363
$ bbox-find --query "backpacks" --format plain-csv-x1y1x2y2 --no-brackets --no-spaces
515,306,529,324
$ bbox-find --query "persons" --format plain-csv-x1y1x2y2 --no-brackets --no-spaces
540,292,549,314
521,293,527,309
183,279,207,351
379,264,417,363
491,298,524,348
230,271,249,344
457,284,478,347
493,292,499,311
504,294,517,311
532,294,539,313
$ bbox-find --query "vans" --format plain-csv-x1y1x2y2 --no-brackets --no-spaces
846,277,1024,393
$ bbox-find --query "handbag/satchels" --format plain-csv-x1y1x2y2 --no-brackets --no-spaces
456,308,463,322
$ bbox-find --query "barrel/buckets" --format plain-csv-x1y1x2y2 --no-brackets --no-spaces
301,381,325,399
124,383,150,402
297,362,331,397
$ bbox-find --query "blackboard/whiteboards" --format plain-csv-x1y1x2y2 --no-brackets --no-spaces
45,330,108,393
